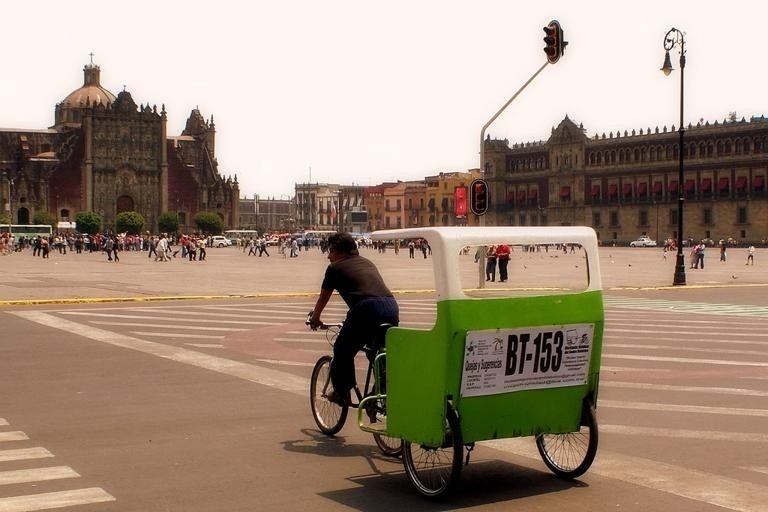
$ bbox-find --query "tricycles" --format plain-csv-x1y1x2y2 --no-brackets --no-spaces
311,226,604,496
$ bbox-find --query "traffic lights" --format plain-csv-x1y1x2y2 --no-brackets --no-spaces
543,20,563,64
470,179,489,216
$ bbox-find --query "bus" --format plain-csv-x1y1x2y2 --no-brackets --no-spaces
305,230,337,240
0,224,52,245
222,230,258,244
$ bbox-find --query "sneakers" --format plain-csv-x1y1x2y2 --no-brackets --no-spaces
328,392,351,405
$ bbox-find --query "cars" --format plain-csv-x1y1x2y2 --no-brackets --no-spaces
630,237,657,247
204,236,232,248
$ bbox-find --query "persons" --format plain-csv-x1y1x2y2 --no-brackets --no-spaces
523,243,577,254
312,231,400,409
662,235,756,269
237,231,432,259
0,229,213,261
484,244,499,281
495,245,513,282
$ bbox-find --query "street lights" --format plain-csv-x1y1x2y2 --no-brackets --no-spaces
660,28,687,285
8,179,14,233
